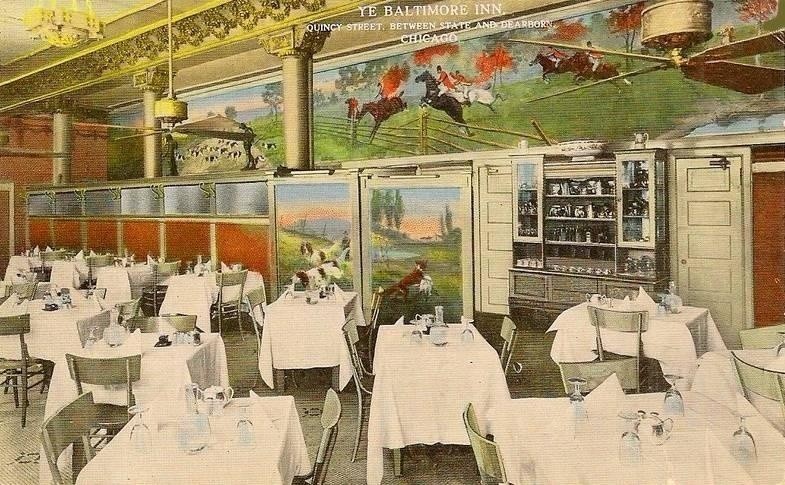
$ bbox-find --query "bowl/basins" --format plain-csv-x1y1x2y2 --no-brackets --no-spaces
560,140,606,162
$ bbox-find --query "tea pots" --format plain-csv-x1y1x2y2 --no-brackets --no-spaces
416,313,433,336
631,131,650,151
584,293,608,307
198,383,234,412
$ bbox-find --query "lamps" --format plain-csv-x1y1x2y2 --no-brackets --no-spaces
19,0,105,52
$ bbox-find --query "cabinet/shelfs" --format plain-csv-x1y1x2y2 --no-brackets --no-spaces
510,151,668,330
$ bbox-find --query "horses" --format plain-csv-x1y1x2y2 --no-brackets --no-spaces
529,52,575,85
571,52,632,86
345,97,360,130
359,91,407,145
446,72,506,114
415,70,475,136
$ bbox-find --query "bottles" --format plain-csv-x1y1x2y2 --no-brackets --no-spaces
104,309,124,348
430,306,448,346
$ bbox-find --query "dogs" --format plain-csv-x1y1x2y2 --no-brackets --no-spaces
260,142,277,151
300,241,328,265
187,139,242,164
174,152,187,164
290,247,351,291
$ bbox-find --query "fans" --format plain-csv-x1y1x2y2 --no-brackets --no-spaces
72,1,256,161
504,0,785,105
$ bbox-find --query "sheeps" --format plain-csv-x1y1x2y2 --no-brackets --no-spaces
419,275,432,296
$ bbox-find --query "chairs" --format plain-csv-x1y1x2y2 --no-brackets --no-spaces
0,245,784,485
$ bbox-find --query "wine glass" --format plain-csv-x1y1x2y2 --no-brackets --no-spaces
730,406,755,460
234,402,257,451
460,318,474,345
664,374,684,418
129,403,151,452
85,326,98,352
409,319,423,346
657,293,667,315
617,411,643,462
568,376,586,421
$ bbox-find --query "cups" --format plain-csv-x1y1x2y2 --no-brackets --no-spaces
517,258,542,268
